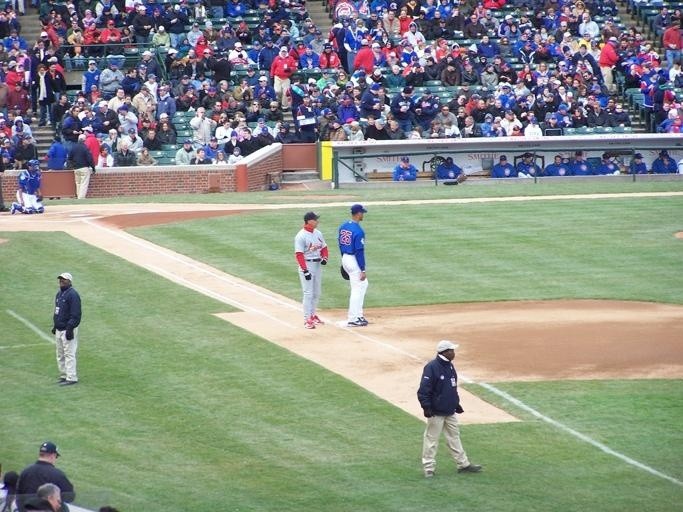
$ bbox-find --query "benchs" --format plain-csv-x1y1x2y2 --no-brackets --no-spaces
363,163,636,182
116,0,682,167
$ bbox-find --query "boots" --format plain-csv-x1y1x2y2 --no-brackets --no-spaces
11,202,22,214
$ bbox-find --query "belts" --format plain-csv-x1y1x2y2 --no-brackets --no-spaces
307,259,321,262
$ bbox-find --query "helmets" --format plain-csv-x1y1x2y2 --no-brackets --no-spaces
28,160,39,172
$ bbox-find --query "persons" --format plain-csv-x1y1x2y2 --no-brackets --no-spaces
51,272,82,385
337,205,369,327
0,442,120,512
295,212,329,328
417,339,482,478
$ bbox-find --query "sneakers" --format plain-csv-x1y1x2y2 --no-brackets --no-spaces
304,315,323,329
348,317,367,326
57,378,77,385
426,464,481,478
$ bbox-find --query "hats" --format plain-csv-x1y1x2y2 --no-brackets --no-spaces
500,150,668,161
40,442,60,456
447,157,453,166
351,204,367,213
304,212,319,220
1,0,680,143
58,273,72,281
437,340,458,353
401,157,409,163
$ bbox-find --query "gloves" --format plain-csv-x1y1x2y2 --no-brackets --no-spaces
321,258,327,265
66,329,73,340
52,328,56,334
424,406,433,417
456,405,464,413
304,270,311,280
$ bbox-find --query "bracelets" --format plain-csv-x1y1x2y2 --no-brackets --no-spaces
362,271,366,273
